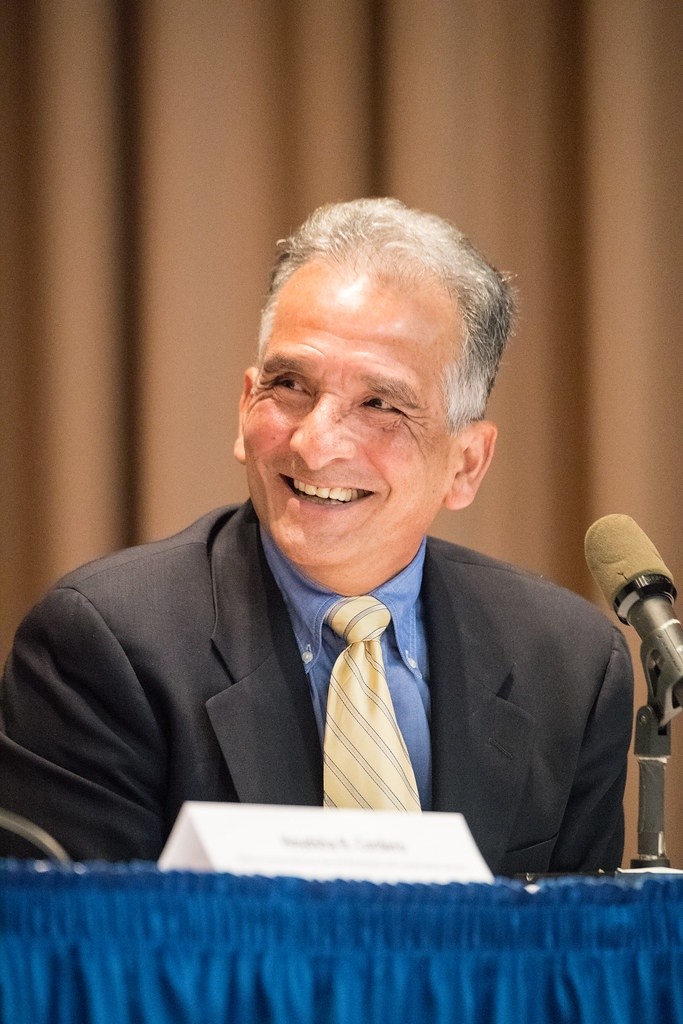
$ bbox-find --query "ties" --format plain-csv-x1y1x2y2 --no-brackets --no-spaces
322,596,421,812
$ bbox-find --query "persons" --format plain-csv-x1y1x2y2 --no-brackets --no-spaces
0,196,631,886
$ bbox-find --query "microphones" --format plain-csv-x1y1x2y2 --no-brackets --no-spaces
583,512,682,728
